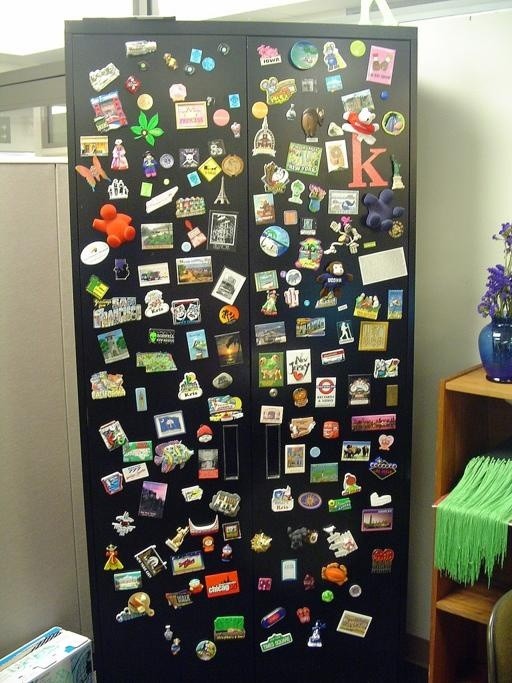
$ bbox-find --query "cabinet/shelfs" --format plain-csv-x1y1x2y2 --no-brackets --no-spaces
426,364,512,682
61,17,419,682
0,152,94,682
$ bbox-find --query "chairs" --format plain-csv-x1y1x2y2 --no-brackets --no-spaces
485,587,512,679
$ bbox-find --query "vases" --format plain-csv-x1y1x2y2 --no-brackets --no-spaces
472,320,511,383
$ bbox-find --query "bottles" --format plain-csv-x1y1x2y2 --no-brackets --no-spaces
478,311,512,384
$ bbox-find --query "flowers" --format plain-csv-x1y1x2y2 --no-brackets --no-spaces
476,220,512,319
